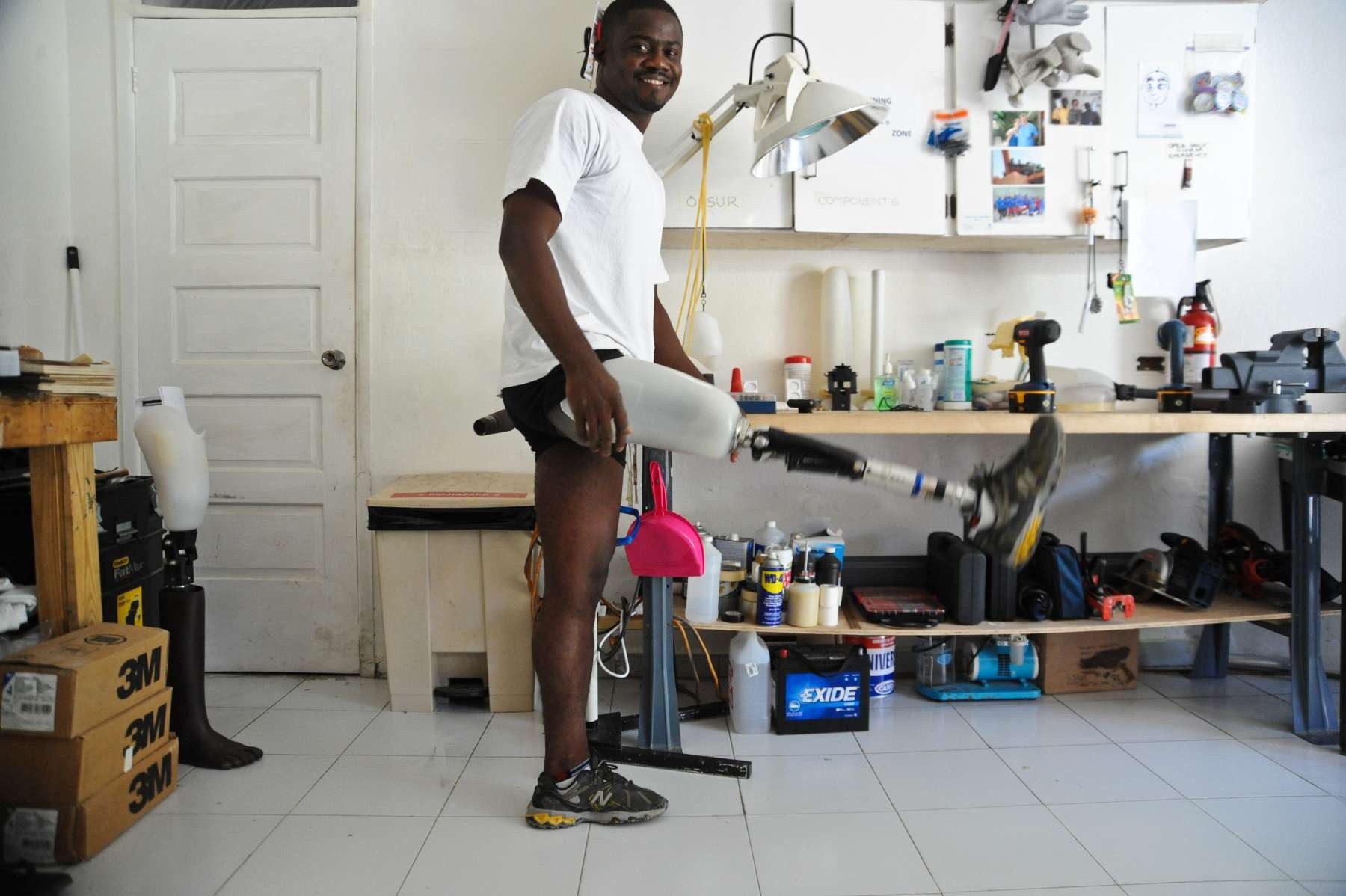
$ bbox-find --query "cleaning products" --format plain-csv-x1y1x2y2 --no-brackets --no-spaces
873,353,900,410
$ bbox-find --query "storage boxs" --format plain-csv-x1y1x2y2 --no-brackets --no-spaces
790,528,846,586
0,733,179,867
1027,627,1139,695
0,686,174,802
0,624,170,739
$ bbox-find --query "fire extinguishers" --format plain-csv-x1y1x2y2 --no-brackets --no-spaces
1176,279,1222,386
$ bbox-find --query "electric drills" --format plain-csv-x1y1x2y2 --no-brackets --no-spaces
984,317,1061,413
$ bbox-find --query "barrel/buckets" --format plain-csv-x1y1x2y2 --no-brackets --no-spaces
728,631,771,735
844,635,898,698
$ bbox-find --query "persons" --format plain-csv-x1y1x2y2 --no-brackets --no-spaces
136,406,268,772
499,0,1064,830
994,96,1102,219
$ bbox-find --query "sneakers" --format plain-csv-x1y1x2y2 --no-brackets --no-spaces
524,756,669,829
962,415,1066,569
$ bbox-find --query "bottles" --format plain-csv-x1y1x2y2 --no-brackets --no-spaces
682,522,710,600
751,554,768,582
815,547,841,586
756,554,784,627
792,546,816,583
787,576,819,627
755,520,786,556
685,535,722,623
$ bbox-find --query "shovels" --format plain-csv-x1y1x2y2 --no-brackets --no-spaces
624,462,706,578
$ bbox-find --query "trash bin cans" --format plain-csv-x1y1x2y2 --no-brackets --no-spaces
370,470,540,712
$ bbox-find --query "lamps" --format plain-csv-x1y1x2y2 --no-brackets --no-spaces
659,33,885,180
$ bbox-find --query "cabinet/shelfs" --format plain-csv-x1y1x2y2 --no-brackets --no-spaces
635,412,1346,748
644,4,948,252
954,2,1253,255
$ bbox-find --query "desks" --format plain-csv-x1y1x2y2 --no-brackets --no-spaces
0,391,119,634
